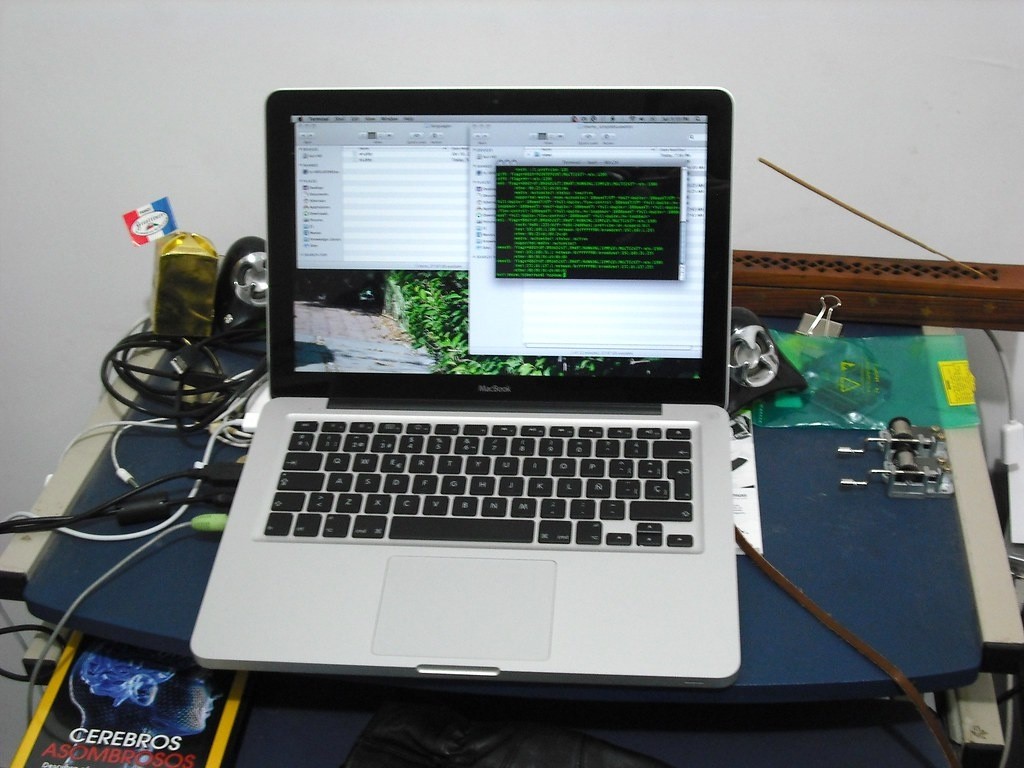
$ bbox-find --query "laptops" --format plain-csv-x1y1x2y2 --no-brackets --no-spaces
189,92,740,687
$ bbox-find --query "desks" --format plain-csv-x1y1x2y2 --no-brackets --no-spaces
0,328,1024,768
